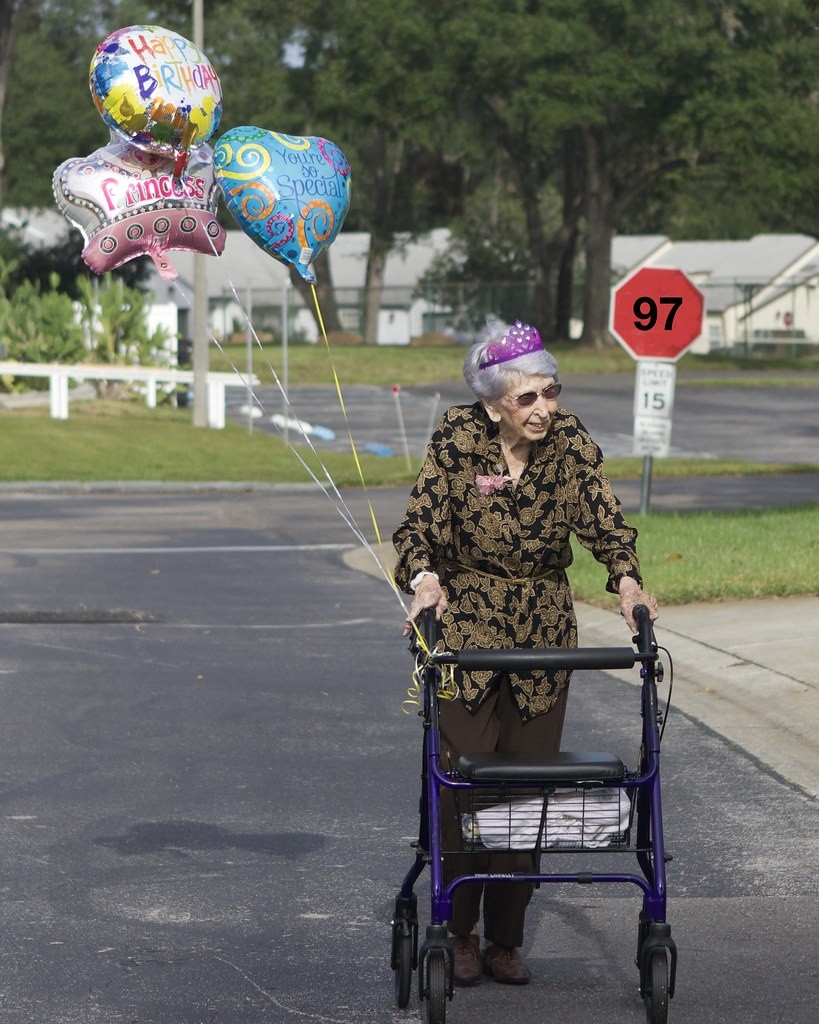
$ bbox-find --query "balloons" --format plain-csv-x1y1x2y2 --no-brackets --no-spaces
89,22,224,181
212,126,353,286
50,126,228,279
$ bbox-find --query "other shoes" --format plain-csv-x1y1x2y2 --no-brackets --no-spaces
480,944,532,985
446,935,482,986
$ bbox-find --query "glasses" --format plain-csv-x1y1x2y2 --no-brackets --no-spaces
500,378,561,406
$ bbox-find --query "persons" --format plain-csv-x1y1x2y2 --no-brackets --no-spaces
388,331,664,989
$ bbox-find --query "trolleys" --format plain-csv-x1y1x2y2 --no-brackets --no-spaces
386,598,678,1024
